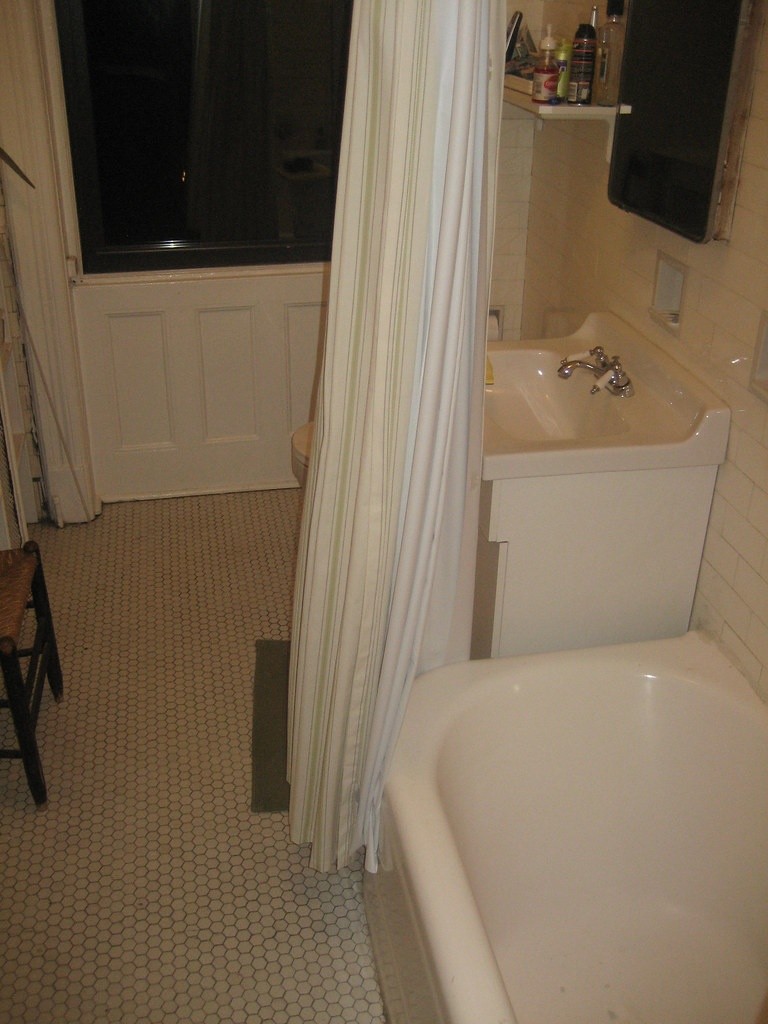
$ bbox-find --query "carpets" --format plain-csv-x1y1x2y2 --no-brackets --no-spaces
250,634,292,813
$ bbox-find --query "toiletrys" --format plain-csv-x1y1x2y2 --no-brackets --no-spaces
530,19,597,110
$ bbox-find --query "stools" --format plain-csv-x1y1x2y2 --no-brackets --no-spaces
1,540,64,814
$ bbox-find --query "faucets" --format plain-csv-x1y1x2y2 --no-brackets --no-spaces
558,344,636,399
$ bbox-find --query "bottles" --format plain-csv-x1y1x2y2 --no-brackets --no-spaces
591,0,625,106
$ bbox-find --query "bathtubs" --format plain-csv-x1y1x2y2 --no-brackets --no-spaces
362,633,768,1020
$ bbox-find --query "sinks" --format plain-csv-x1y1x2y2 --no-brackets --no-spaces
461,311,732,482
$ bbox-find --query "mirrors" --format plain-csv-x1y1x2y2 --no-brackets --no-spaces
605,0,767,245
